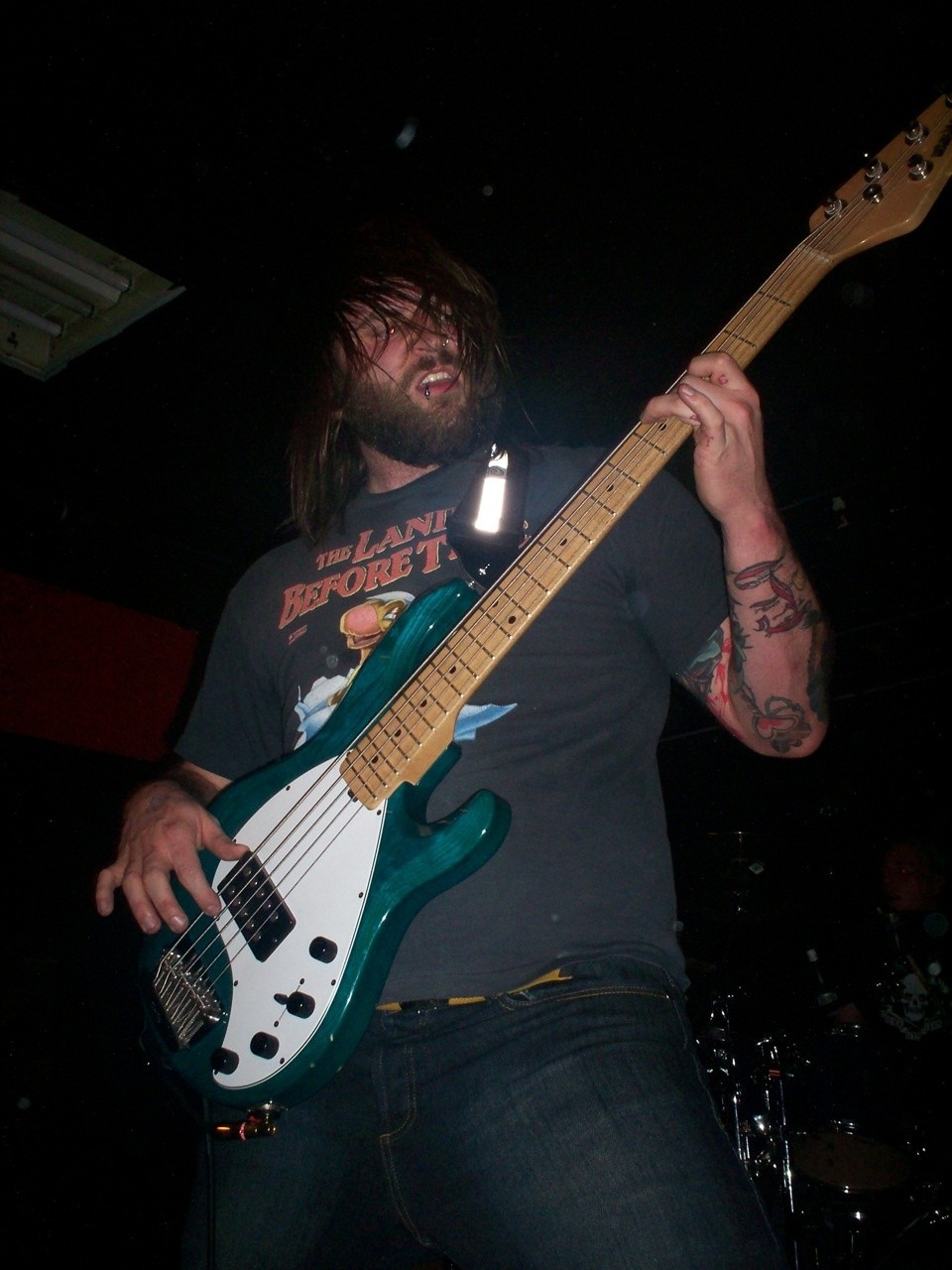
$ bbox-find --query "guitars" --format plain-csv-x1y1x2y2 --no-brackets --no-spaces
140,86,952,1105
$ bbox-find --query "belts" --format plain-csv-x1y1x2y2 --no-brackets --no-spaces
379,966,572,1013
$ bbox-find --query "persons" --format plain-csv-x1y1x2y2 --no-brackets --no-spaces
95,228,826,1270
835,840,952,1140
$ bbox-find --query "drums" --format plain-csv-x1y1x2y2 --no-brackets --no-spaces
785,1020,929,1196
